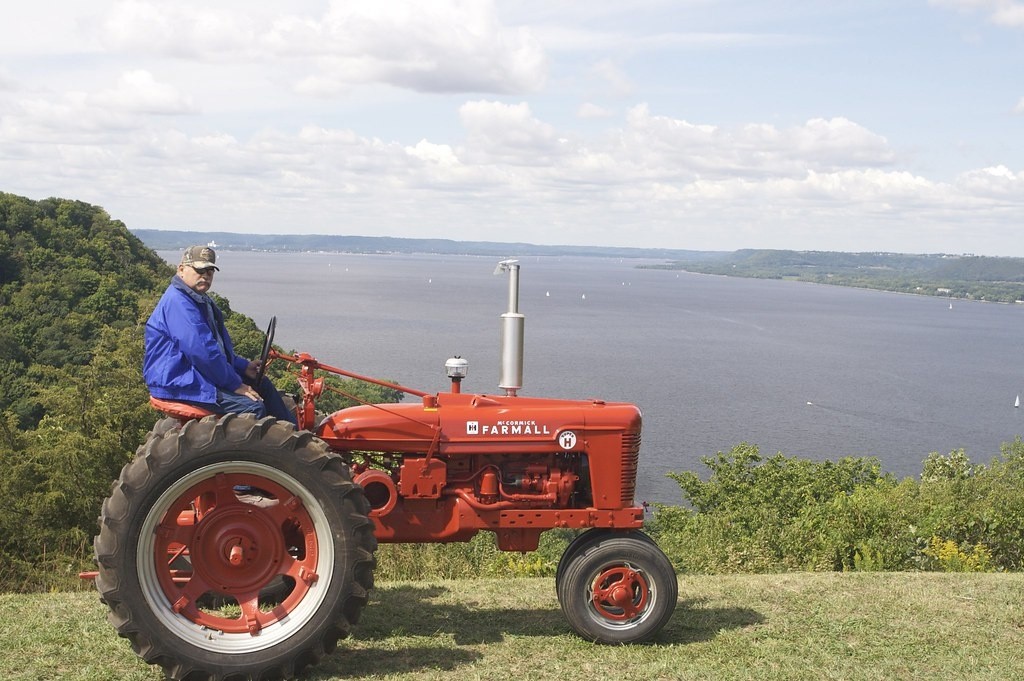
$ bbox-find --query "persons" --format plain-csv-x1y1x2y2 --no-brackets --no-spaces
143,245,298,493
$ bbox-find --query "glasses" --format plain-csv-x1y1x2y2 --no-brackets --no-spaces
191,266,215,275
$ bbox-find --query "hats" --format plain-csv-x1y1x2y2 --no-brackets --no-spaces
182,245,219,272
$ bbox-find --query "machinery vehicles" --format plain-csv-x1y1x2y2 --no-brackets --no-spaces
95,260,680,680
